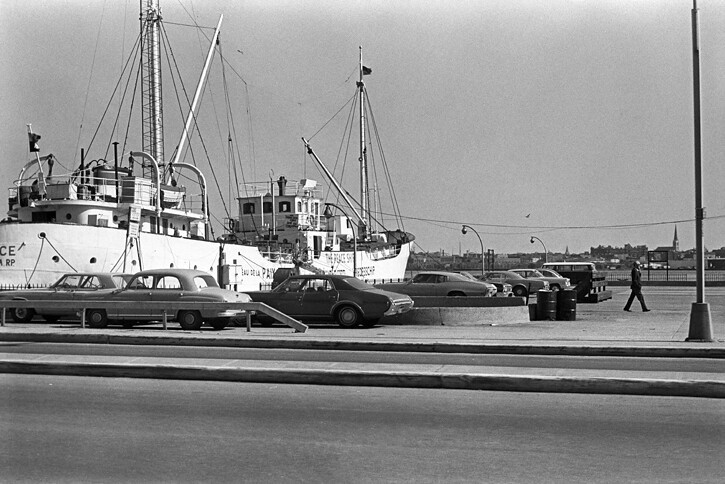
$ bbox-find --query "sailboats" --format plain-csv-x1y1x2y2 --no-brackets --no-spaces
0,0,416,291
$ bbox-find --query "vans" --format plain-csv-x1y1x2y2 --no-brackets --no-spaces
536,269,563,278
537,261,597,286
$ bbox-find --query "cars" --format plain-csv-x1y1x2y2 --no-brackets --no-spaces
485,270,551,297
0,264,257,331
508,268,571,293
371,269,496,298
235,274,414,328
445,271,515,298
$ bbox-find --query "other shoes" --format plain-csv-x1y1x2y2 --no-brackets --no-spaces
643,309,650,311
624,308,632,312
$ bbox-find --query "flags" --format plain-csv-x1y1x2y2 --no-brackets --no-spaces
27,133,41,152
363,66,372,75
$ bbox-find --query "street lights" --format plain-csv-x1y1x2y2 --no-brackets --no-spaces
461,224,485,276
530,235,548,263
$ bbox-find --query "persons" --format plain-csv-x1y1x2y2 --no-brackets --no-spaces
624,261,650,312
396,229,408,243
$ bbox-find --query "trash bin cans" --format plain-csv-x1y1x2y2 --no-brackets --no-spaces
537,290,557,321
557,290,577,321
